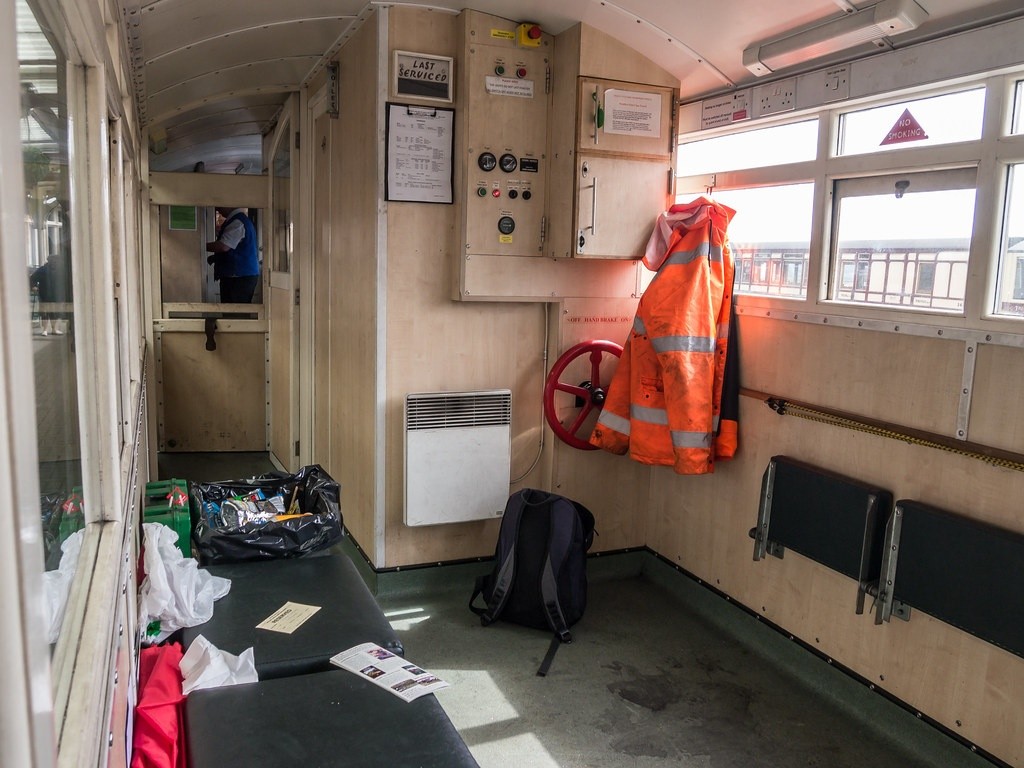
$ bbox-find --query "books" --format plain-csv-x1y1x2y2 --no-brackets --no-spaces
328,641,451,704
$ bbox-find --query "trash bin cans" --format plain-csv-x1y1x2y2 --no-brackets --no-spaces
190,476,331,566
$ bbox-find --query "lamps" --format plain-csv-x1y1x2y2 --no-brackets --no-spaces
743,0,929,78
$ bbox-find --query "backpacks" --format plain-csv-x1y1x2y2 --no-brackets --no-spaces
468,488,600,677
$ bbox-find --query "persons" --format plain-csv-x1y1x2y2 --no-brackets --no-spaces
31,255,64,335
206,206,260,320
